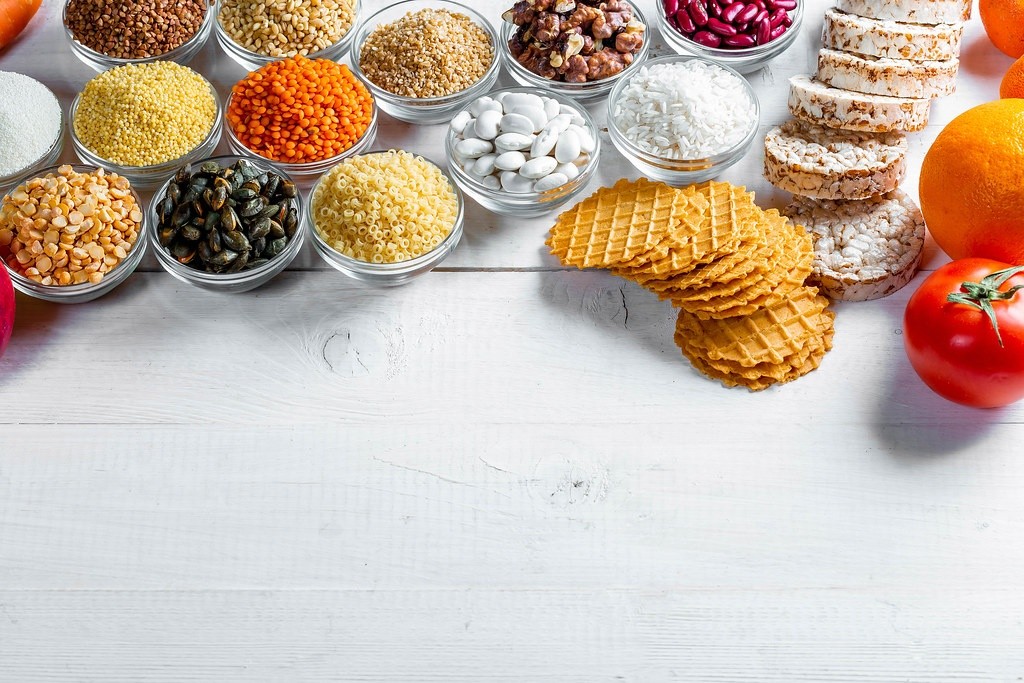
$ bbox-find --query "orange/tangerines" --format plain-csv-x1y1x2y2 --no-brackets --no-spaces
918,0,1024,271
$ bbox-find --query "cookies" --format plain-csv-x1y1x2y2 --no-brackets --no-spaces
544,176,835,390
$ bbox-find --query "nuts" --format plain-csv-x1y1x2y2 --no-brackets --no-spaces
502,0,647,83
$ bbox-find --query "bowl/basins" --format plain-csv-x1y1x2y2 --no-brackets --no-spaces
445,86,601,219
221,58,380,188
68,64,223,191
304,149,466,288
146,154,305,295
349,0,503,126
499,0,653,108
652,0,805,75
211,0,362,72
60,0,213,73
605,54,762,186
0,69,66,194
0,163,147,305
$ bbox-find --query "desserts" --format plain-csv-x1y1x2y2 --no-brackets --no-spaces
764,0,973,303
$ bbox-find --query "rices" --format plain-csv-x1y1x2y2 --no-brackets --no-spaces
614,60,755,160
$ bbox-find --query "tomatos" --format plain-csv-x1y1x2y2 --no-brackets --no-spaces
901,258,1024,408
0,0,42,52
